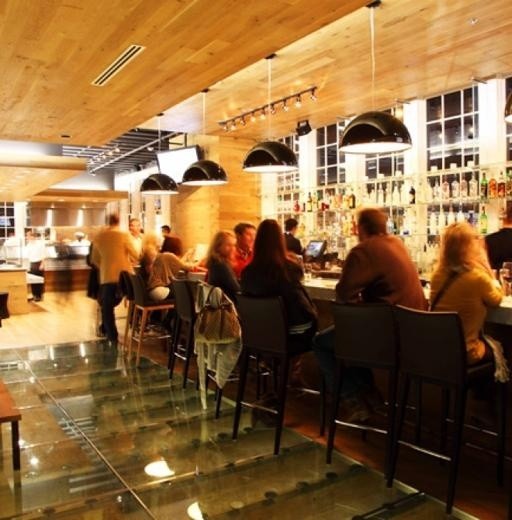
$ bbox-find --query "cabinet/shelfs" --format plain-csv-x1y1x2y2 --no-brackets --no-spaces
303,161,512,266
0,267,30,317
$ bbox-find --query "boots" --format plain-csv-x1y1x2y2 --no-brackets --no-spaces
470,400,494,426
159,308,176,332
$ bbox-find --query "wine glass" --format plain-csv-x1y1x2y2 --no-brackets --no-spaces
501,262,512,298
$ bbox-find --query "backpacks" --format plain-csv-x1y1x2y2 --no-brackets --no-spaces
279,283,321,354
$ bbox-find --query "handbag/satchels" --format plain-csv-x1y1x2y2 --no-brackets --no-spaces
193,284,242,345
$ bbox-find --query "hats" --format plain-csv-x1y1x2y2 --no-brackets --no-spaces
45,228,50,232
76,232,84,237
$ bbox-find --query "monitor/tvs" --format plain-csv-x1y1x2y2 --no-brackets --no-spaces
304,239,327,260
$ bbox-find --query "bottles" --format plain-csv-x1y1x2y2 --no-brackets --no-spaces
292,171,512,213
410,240,438,274
344,215,356,237
498,209,505,230
400,210,487,236
387,218,393,235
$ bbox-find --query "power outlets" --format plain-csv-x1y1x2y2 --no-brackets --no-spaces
243,52,306,173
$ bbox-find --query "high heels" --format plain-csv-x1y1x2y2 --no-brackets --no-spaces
251,407,280,428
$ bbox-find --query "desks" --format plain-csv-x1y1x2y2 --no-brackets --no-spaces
0,381,22,470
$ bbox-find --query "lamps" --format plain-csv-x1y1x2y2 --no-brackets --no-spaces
140,111,178,195
215,85,319,133
181,88,229,188
335,0,414,155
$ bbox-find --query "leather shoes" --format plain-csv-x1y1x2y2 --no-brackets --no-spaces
336,397,371,430
28,294,41,301
363,390,388,417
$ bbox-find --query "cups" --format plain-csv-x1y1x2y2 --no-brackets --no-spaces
304,273,312,282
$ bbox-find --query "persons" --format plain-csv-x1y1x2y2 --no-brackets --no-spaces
2,225,92,303
238,217,323,393
87,212,139,345
147,236,196,341
430,217,511,466
159,225,172,239
125,217,145,330
310,206,428,433
482,199,511,286
206,230,240,308
198,221,259,285
281,217,312,262
87,241,123,338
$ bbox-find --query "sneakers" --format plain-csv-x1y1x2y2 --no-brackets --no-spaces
97,327,118,343
